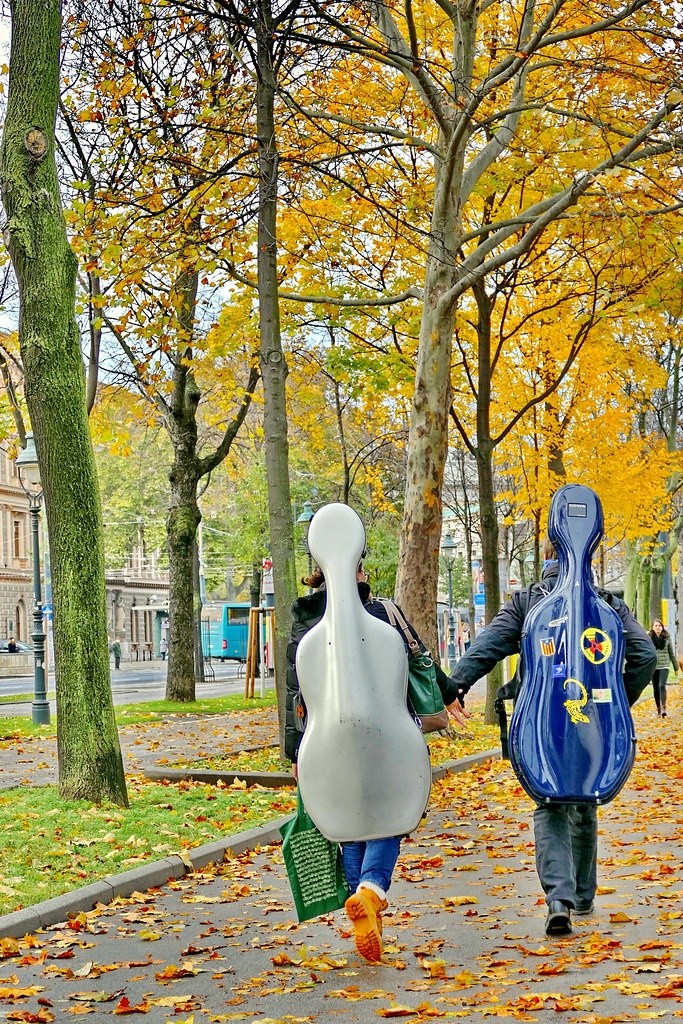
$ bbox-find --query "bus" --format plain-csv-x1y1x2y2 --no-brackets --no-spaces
199,601,273,664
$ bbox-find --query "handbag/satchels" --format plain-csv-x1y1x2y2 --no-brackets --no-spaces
379,601,449,733
278,778,352,923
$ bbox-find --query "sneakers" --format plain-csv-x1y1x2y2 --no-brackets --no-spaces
572,901,594,914
345,885,388,963
545,900,573,936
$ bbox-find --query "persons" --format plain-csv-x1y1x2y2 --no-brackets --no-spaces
282,553,472,961
160,638,168,660
647,619,679,718
448,539,657,940
8,637,20,653
462,622,471,650
109,638,122,670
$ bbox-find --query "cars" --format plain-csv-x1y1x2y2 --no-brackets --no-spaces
0,640,33,653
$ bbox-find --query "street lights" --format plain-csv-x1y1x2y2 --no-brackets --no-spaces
441,529,459,671
15,430,51,726
296,499,318,594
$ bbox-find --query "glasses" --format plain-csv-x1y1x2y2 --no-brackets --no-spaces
360,568,370,583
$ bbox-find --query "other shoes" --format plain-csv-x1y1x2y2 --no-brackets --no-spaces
661,705,667,718
657,705,661,717
